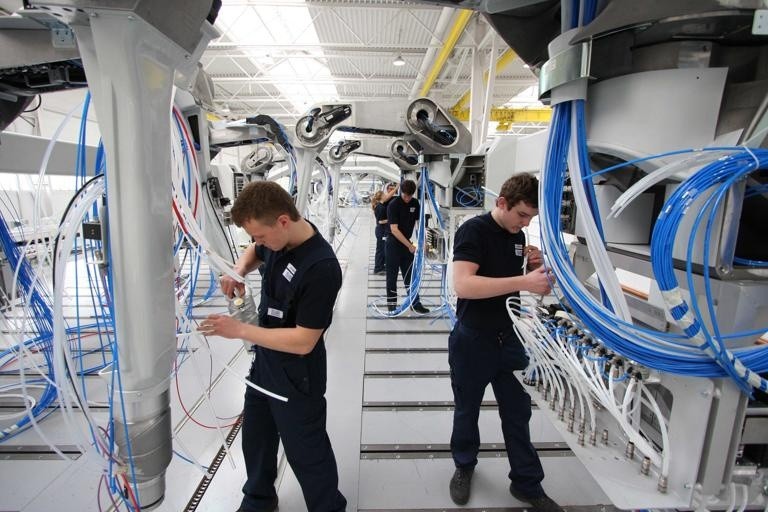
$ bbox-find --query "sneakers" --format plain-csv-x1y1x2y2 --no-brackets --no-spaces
450,467,473,506
510,481,565,512
388,304,397,313
411,303,430,313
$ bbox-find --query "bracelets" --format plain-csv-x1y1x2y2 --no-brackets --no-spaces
408,242,414,247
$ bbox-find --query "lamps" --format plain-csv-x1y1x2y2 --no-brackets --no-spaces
393,56,406,66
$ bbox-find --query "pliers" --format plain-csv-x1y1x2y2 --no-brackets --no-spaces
230,292,246,313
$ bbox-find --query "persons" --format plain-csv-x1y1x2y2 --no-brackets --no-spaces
450,174,564,512
372,182,400,274
196,181,348,512
384,184,398,267
385,180,430,313
